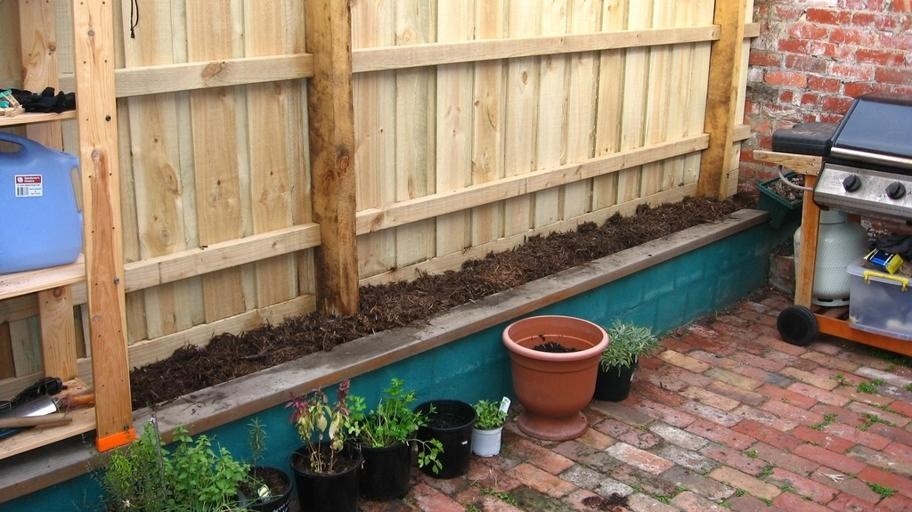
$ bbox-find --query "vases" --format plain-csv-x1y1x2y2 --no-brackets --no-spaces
501,314,610,443
413,398,478,479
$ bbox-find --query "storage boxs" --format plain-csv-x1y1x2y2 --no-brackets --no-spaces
756,170,804,234
845,250,912,342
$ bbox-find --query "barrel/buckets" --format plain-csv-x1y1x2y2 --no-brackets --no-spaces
1,131,84,276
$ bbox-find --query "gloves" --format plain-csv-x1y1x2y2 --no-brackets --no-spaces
13,87,75,112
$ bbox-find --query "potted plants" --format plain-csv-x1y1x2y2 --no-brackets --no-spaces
86,377,293,510
594,318,659,402
469,400,509,458
292,378,447,511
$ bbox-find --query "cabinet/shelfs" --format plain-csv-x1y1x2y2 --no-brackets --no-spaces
1,0,138,461
